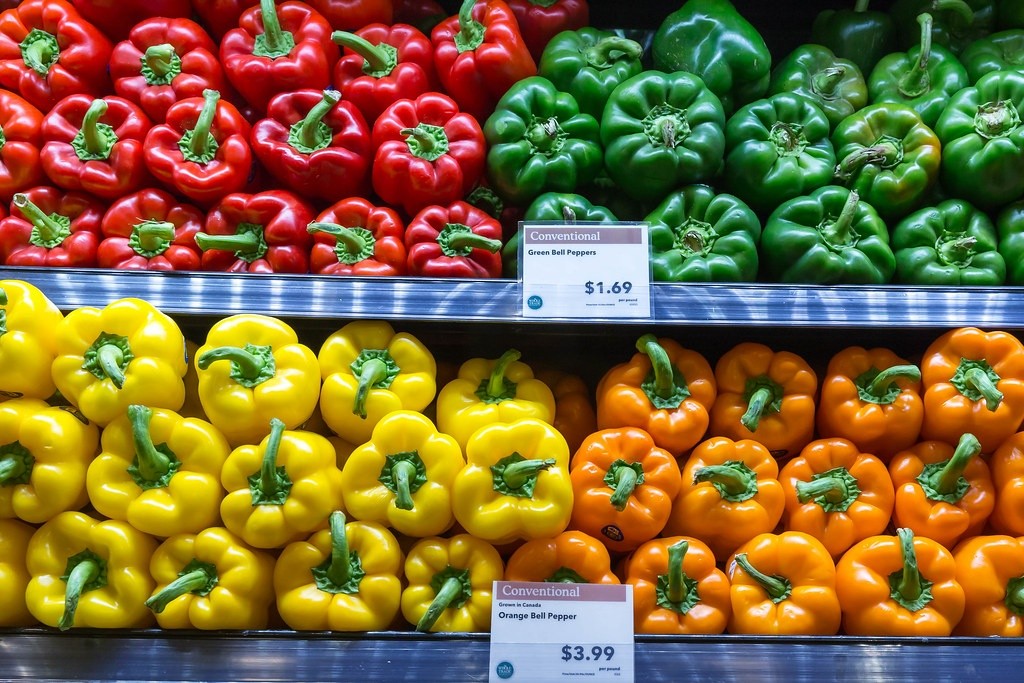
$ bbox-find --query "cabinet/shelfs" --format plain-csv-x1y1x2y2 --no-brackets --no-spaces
0,265,1024,683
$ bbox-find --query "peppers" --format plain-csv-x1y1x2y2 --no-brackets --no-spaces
0,0,1024,638
